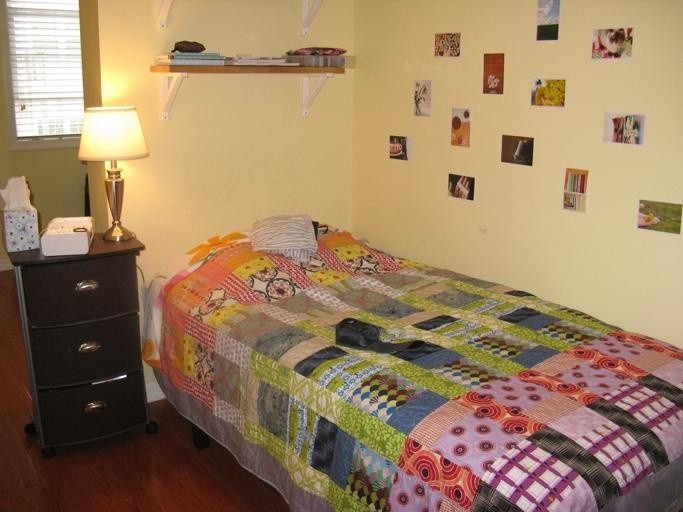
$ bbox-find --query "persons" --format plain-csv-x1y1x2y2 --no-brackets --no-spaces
448,177,470,199
639,203,660,227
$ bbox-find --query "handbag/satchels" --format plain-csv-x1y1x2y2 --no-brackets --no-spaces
335,318,424,354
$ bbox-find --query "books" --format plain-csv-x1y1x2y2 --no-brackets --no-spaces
158,50,300,67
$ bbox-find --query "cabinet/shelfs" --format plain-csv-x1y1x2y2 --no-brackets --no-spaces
7,230,150,458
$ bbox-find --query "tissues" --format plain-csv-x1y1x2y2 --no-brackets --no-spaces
0,174,40,252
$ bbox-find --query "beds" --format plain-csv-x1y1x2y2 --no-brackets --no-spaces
142,215,682,511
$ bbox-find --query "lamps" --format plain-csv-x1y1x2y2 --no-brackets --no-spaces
77,106,149,243
